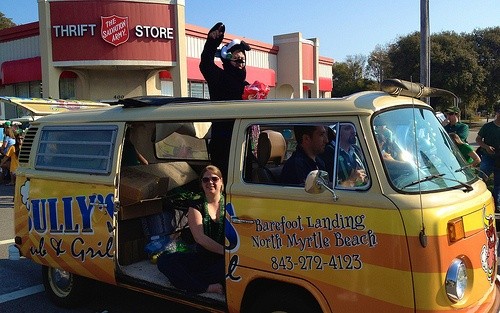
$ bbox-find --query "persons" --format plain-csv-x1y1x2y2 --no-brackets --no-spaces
375,122,420,176
0,122,22,176
475,101,500,213
441,106,469,142
0,132,25,202
320,121,370,187
200,22,252,183
449,132,481,175
278,125,367,188
156,165,227,295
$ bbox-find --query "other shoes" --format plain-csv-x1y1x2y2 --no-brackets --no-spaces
4,182,14,186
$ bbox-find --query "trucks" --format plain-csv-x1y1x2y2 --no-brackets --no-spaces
1,77,500,313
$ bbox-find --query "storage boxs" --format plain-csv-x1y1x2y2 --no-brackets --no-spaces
119,176,169,221
120,166,162,201
131,162,201,193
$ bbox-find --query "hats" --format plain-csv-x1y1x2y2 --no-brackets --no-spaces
493,100,500,113
444,106,460,114
4,122,10,126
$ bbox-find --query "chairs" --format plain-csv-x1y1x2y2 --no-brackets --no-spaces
258,130,287,185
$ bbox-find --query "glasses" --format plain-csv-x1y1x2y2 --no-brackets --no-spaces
200,177,220,183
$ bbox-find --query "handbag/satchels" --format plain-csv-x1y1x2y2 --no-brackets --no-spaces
157,240,200,290
